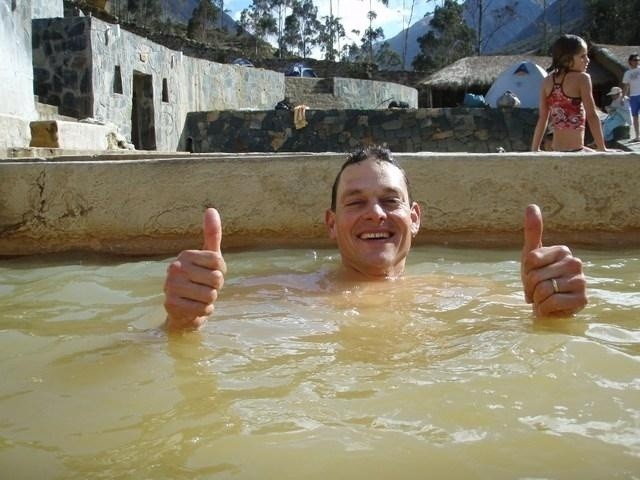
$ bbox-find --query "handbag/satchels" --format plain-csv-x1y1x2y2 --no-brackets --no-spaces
388,100,409,109
496,90,521,108
462,93,491,108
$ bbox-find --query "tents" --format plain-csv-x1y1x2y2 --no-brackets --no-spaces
484,61,547,109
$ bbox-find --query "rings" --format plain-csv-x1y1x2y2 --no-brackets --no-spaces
551,278,560,294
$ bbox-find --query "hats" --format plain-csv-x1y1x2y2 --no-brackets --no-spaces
606,87,623,95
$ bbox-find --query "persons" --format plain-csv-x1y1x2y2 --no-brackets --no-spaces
531,35,625,153
585,86,633,147
149,145,588,339
620,54,640,143
